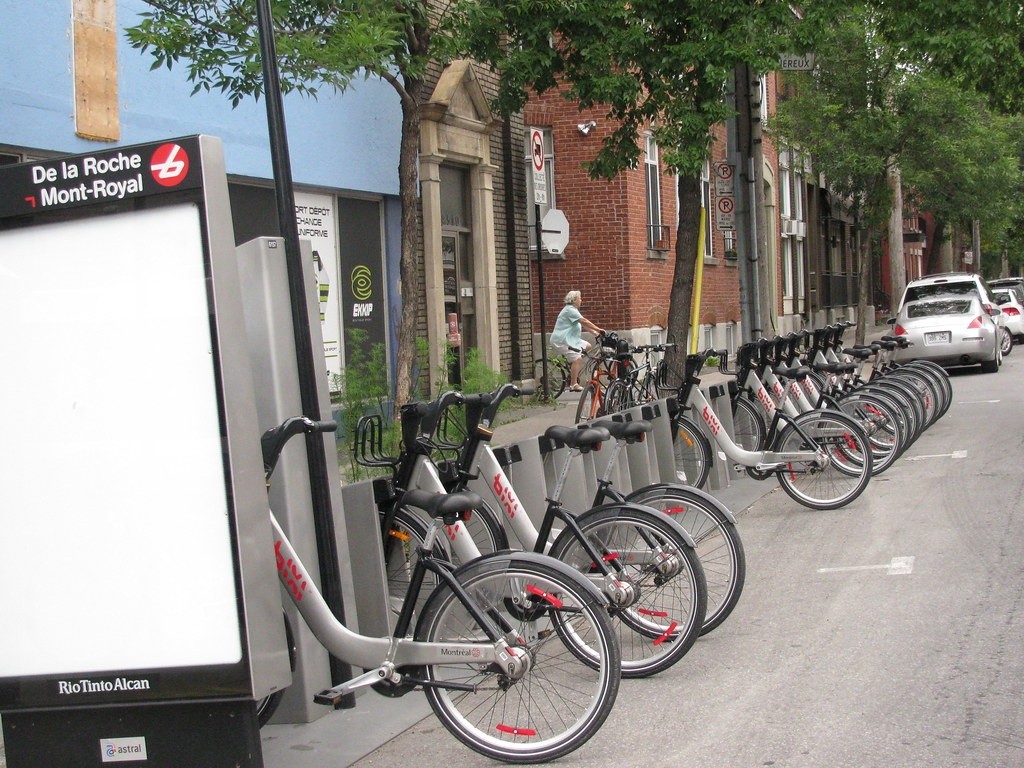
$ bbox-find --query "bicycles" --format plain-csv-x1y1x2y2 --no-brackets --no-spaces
418,382,748,641
353,391,709,679
254,416,622,766
533,331,676,425
656,320,953,511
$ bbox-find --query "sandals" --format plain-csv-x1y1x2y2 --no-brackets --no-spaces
570,383,584,391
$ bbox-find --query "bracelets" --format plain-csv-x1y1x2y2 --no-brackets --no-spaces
596,328,599,331
591,330,596,334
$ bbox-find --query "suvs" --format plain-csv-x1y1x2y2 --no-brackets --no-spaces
895,271,1013,357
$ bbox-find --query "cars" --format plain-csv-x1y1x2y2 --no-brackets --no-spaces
986,277,1024,345
886,293,1002,373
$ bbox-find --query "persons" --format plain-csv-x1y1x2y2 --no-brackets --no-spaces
550,291,606,392
